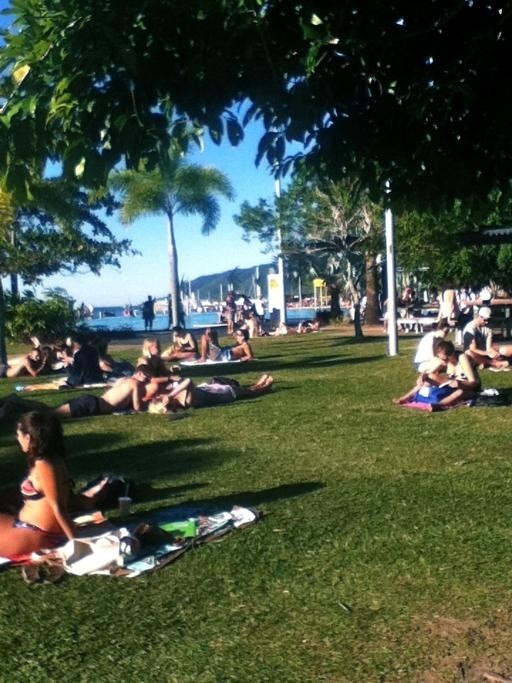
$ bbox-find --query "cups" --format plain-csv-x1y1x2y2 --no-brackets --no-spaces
118,495,132,514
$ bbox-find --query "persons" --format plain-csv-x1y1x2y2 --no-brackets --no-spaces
2,288,323,417
350,276,512,411
0,410,79,562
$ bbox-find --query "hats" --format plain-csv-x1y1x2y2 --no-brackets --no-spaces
478,306,493,321
